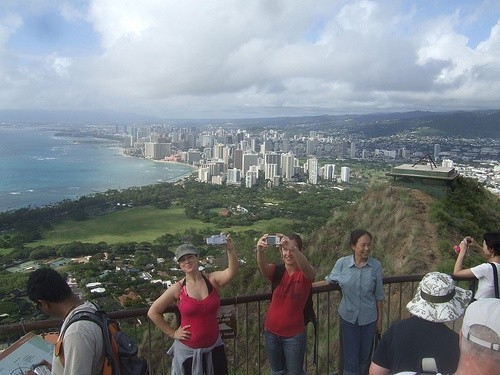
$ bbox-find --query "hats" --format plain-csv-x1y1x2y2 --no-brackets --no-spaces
461,298,500,351
405,271,473,323
175,244,200,262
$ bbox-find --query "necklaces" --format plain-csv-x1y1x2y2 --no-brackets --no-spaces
61,299,82,322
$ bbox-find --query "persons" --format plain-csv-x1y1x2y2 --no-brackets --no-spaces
324,229,383,375
24,268,112,375
394,298,500,375
453,232,500,298
368,271,472,375
146,232,237,375
256,232,315,375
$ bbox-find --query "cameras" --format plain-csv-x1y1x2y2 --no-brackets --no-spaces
466,238,472,244
264,235,281,245
205,235,227,246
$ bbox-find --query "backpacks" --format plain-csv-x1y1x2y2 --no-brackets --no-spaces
54,300,149,375
